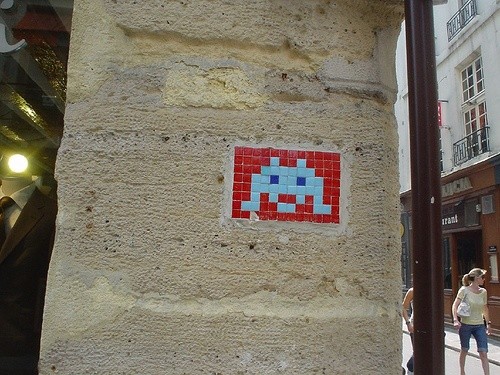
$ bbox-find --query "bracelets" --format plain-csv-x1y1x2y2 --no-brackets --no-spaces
406,320,411,326
487,320,491,325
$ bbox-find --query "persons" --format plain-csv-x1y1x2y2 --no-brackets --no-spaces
402,285,416,372
452,267,492,375
0,167,58,375
